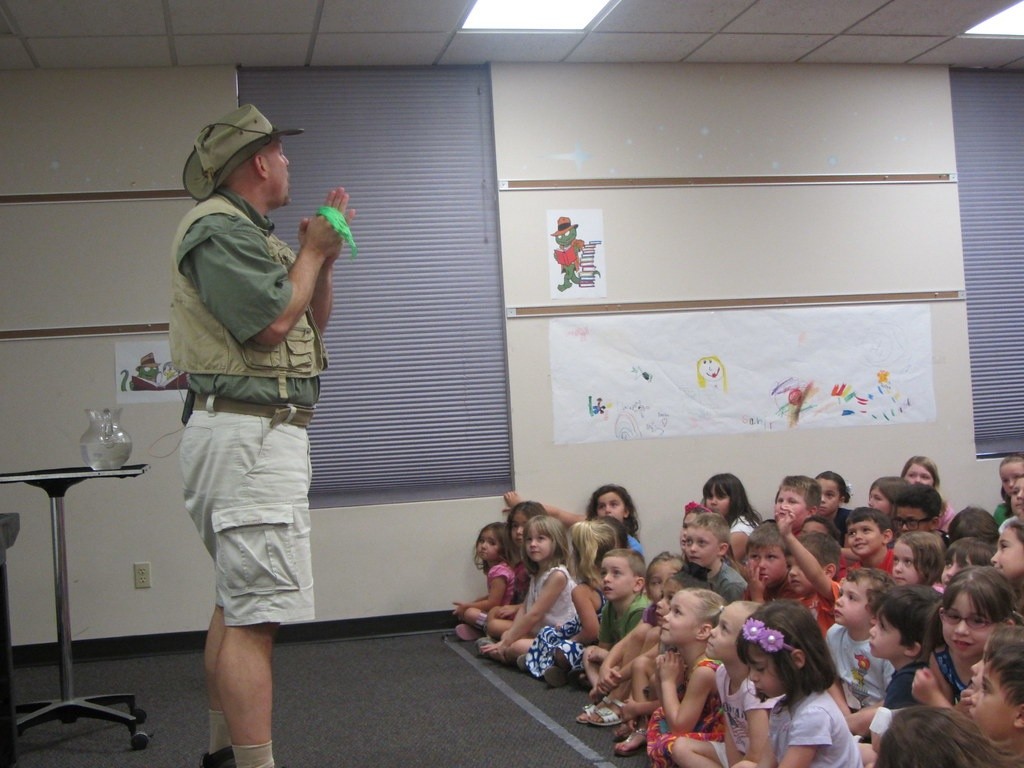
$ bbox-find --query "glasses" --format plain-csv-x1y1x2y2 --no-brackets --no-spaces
943,609,994,630
892,517,932,531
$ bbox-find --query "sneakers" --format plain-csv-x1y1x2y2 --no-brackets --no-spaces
454,623,587,690
199,745,238,768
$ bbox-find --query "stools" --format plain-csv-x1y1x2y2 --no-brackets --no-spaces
0,510,20,768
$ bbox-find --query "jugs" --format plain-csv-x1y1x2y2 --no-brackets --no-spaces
79,405,132,472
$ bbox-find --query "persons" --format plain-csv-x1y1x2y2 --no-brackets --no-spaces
728,597,865,768
576,549,658,726
164,105,357,768
910,565,1023,748
669,600,786,768
647,586,738,768
854,588,950,767
819,568,910,750
970,624,1024,726
873,703,1019,768
448,443,1024,660
610,576,715,761
483,515,572,681
966,639,1024,768
543,520,620,689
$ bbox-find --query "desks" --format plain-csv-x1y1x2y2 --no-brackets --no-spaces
2,459,156,751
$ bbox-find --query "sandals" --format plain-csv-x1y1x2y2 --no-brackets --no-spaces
575,695,647,758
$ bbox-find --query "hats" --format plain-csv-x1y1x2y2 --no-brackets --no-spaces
182,104,305,202
